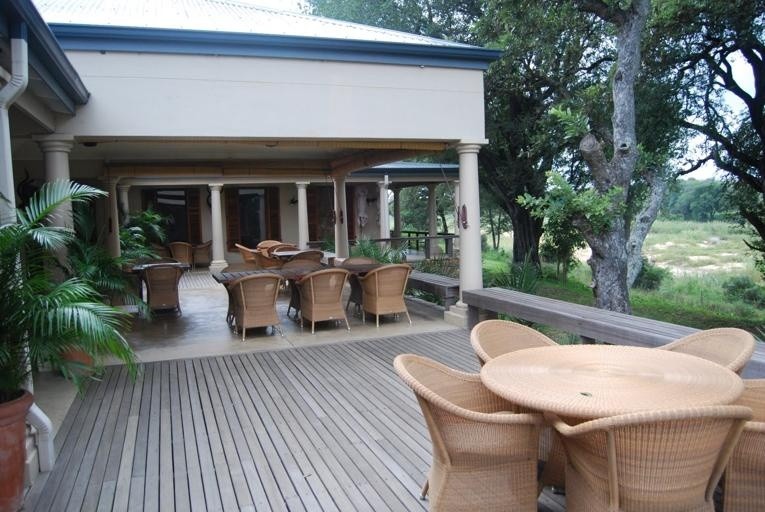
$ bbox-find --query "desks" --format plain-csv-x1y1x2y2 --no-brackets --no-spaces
481,344,744,499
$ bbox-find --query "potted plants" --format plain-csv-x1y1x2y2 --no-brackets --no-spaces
0,179,146,511
38,218,151,379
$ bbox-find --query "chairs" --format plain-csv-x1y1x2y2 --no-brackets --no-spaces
543,405,752,512
470,319,559,367
130,239,413,343
392,353,542,512
722,379,764,512
653,327,756,375
